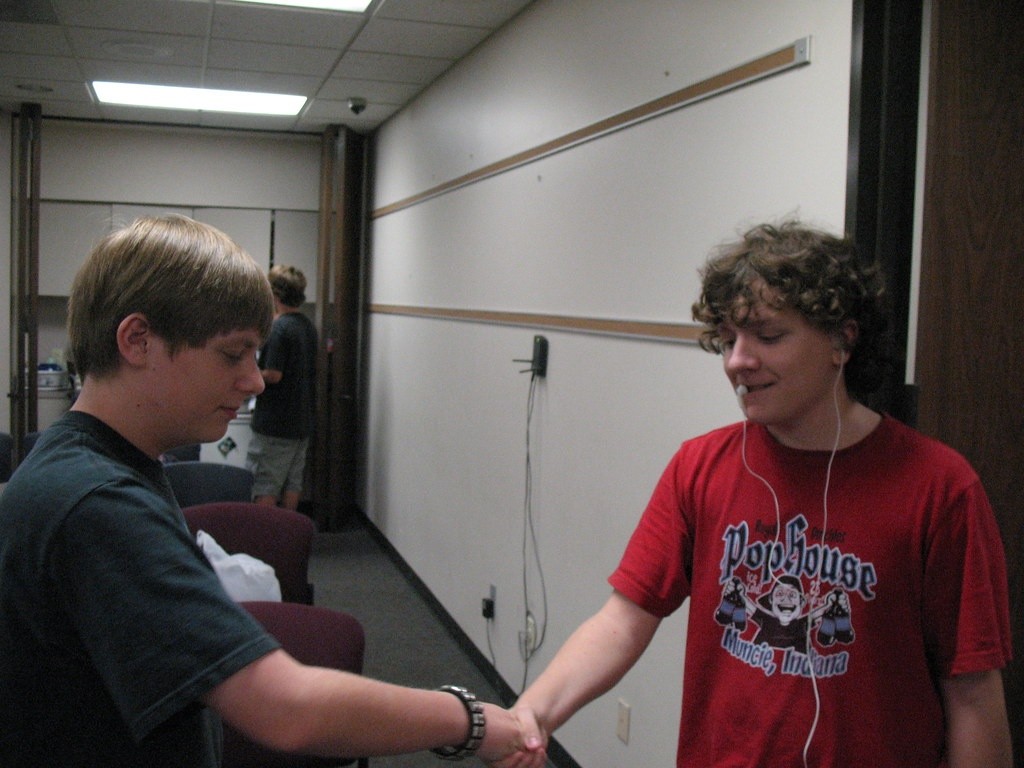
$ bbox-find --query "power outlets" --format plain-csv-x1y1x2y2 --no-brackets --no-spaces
489,585,497,618
524,617,535,650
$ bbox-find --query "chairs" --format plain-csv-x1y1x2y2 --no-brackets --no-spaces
163,461,255,510
22,431,42,461
181,502,314,607
0,431,12,483
221,600,369,768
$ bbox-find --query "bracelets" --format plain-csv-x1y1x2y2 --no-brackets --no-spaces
427,683,486,762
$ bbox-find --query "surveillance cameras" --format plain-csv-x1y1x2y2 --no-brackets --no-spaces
347,98,366,115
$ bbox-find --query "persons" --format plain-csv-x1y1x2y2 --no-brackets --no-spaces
245,262,319,511
0,214,547,768
489,221,1016,768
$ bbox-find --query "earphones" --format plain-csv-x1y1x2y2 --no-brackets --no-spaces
736,385,747,417
832,335,845,360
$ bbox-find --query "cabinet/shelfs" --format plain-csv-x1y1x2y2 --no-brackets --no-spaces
25,200,335,305
